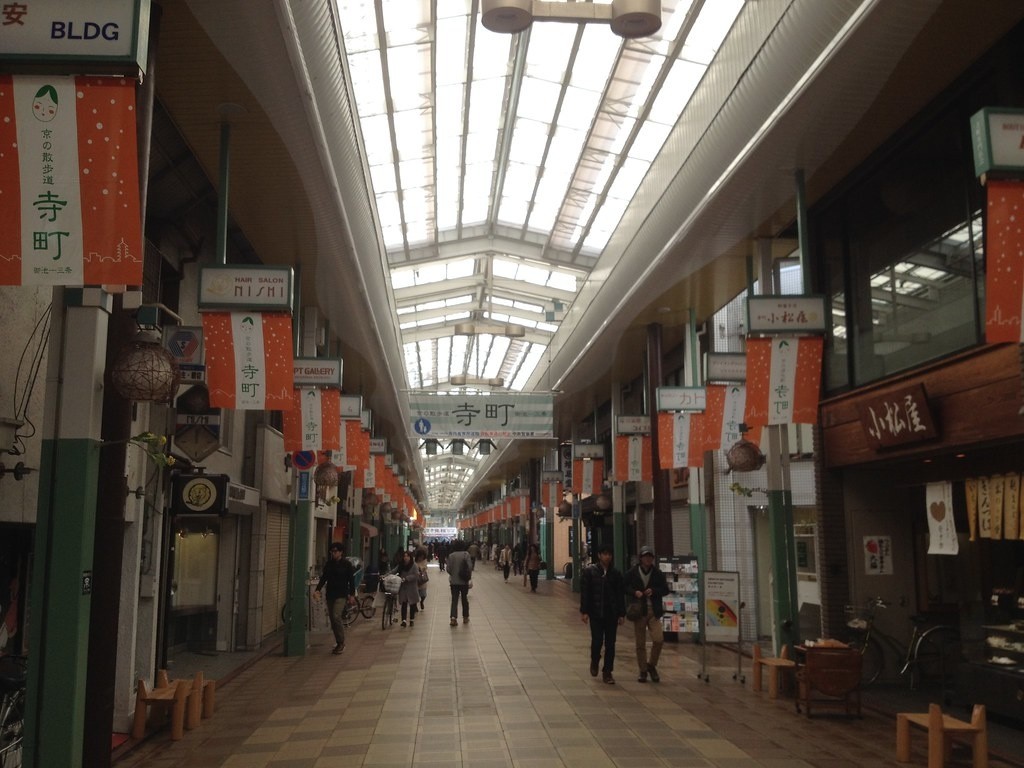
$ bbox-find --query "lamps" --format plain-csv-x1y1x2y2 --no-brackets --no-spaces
313,450,339,487
110,317,181,405
726,422,765,472
363,488,412,524
557,483,612,515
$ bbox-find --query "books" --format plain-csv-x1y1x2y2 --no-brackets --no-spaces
658,560,699,632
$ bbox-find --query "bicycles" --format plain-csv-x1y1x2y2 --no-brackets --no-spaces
378,576,404,630
341,582,376,626
842,597,962,692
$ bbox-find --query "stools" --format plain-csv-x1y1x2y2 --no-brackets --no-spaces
751,643,799,701
133,668,216,744
896,702,989,768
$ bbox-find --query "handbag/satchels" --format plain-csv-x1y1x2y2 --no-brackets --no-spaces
625,602,644,621
524,567,528,587
416,567,429,585
382,573,401,594
458,550,471,582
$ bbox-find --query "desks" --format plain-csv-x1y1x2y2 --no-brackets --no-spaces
791,644,866,722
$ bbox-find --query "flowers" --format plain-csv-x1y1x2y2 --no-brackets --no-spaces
92,431,174,467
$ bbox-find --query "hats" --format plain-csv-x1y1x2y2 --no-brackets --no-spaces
640,545,654,558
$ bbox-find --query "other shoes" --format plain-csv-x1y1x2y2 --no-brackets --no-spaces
590,659,599,677
450,619,458,626
602,670,615,684
463,617,470,624
638,671,648,683
420,601,424,610
332,641,346,654
531,589,536,593
410,621,414,627
647,663,660,682
415,606,418,612
504,578,508,582
401,622,407,627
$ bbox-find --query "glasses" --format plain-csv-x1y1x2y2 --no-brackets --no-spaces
328,549,341,553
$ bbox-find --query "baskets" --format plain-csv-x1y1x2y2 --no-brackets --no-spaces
379,579,402,595
842,605,871,634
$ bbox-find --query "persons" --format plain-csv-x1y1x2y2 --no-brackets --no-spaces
524,544,540,593
580,544,625,684
313,543,355,653
623,546,670,682
447,541,472,625
382,551,419,626
378,546,405,592
446,540,488,570
415,550,428,612
423,539,447,572
495,544,512,583
512,544,523,576
